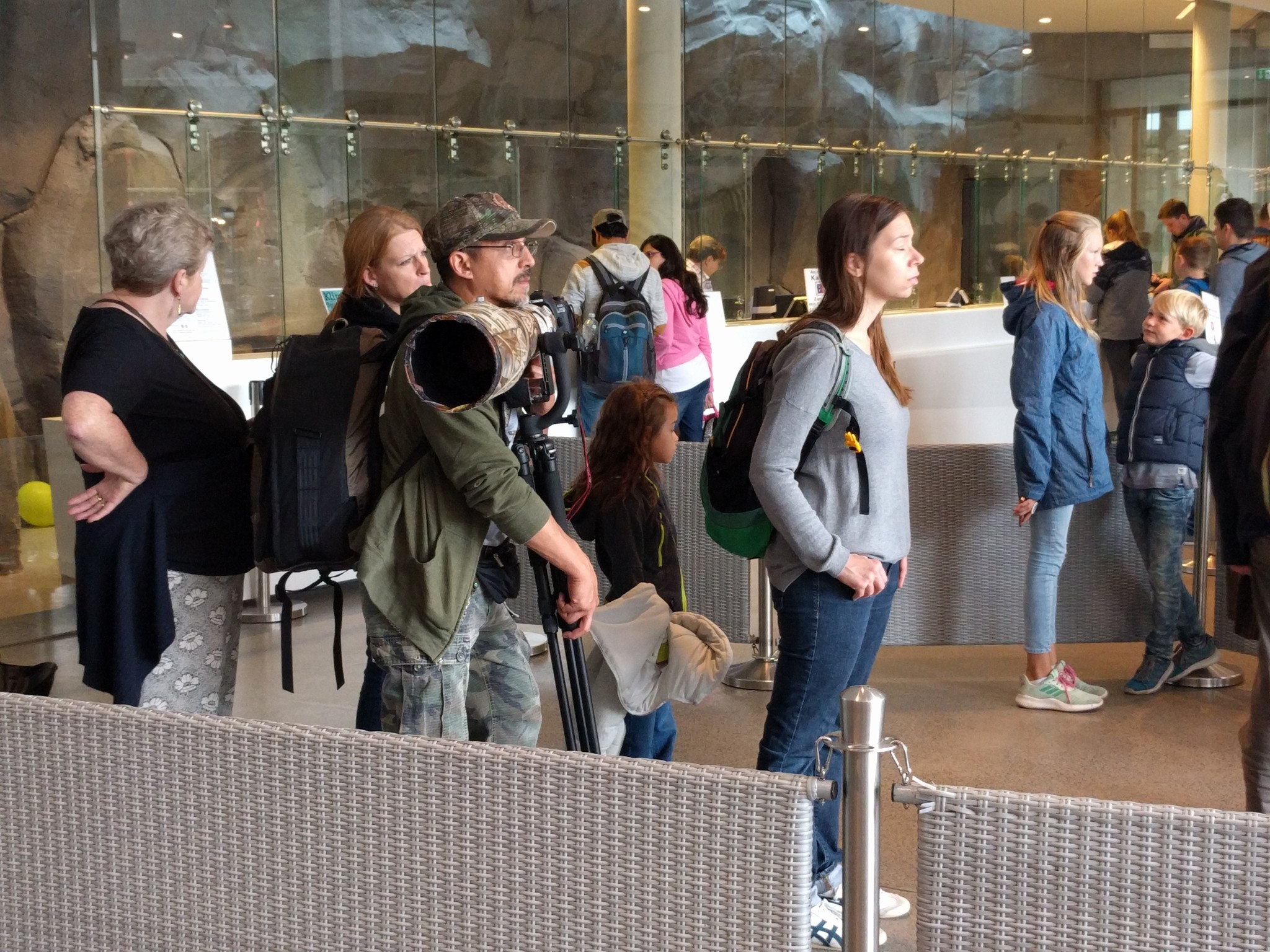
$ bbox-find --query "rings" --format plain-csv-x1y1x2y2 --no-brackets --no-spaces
95,494,102,500
99,501,105,507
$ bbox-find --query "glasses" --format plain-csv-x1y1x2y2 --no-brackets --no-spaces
464,240,539,258
646,251,663,258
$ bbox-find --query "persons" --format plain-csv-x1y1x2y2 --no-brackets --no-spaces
1085,198,1270,815
683,235,727,292
562,379,687,763
347,190,600,748
319,206,433,732
639,234,714,442
560,208,668,438
1002,209,1116,712
62,199,257,717
748,192,925,951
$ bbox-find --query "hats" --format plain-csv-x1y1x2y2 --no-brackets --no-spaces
591,208,628,247
422,191,557,263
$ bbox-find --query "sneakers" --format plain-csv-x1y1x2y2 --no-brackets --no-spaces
1123,652,1174,695
810,898,887,950
1164,632,1220,683
1015,668,1104,713
824,864,911,918
1056,659,1108,700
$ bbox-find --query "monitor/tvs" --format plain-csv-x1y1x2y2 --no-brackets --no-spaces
751,284,777,320
783,296,807,318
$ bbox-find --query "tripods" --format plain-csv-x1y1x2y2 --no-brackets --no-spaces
500,433,602,755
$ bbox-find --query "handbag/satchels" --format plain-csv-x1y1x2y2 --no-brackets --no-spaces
478,536,522,606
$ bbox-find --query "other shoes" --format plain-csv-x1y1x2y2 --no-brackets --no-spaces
1109,435,1119,448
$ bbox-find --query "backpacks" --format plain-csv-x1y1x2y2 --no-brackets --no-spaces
700,318,851,560
250,312,441,573
582,254,656,396
